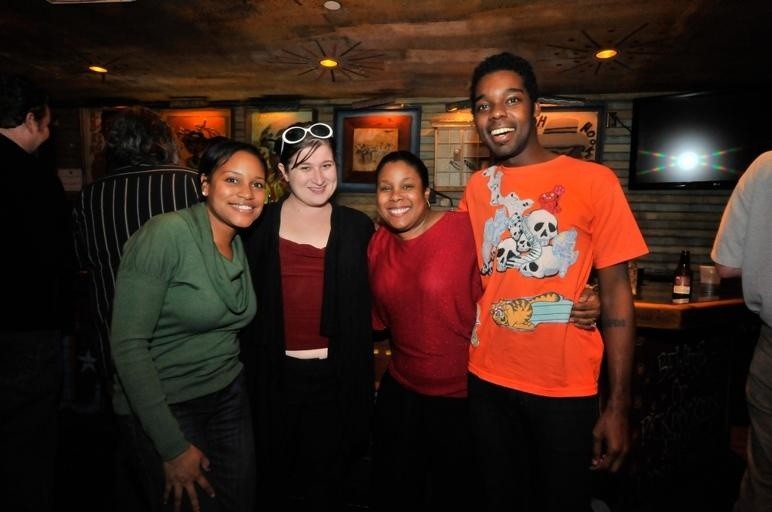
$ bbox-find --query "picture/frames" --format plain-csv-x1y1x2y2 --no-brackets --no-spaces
536,103,608,164
157,104,422,209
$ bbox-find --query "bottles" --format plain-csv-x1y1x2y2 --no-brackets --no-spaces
672,248,691,303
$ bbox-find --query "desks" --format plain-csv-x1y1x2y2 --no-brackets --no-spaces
628,279,745,440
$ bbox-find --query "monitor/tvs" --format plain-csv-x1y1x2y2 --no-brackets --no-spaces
628,92,772,190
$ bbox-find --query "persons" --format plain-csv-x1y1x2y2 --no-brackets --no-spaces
710,150,772,512
465,54,651,512
71,108,207,388
238,122,376,512
0,76,74,512
367,151,601,512
109,143,269,512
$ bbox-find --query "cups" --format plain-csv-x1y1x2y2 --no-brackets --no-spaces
700,265,720,296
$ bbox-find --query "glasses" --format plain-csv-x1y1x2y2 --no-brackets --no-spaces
280,122,335,157
